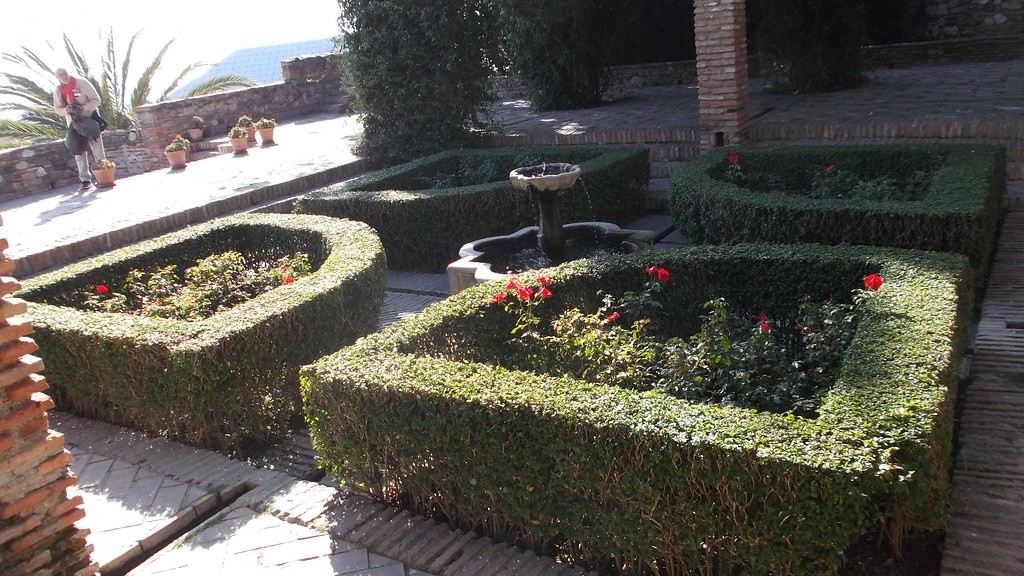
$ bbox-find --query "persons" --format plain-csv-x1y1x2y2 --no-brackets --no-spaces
52,68,106,188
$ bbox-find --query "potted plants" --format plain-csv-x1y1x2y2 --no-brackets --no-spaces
228,127,249,154
184,116,205,142
163,142,187,170
234,114,257,142
172,135,190,162
91,159,116,188
256,116,276,145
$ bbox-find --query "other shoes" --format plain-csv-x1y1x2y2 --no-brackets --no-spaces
81,181,93,188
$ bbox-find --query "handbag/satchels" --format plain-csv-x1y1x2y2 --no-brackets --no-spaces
65,116,102,154
92,110,108,131
